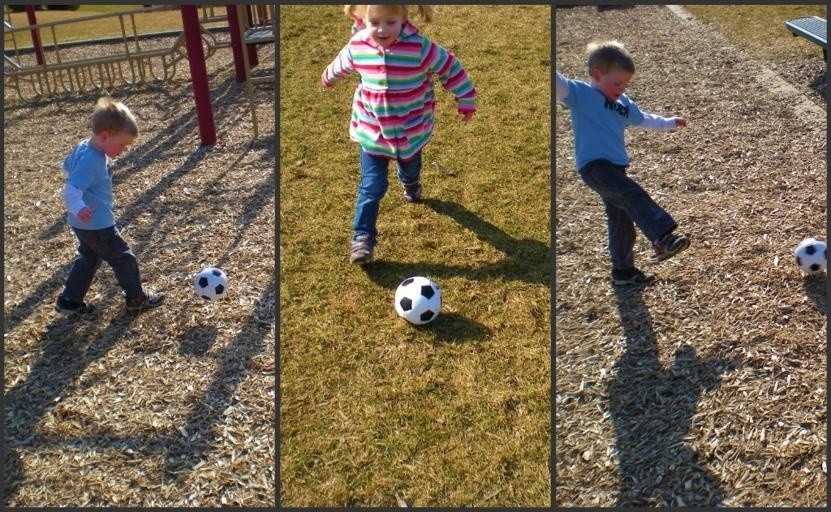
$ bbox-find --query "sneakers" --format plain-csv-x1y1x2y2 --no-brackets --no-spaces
348,235,375,265
612,267,654,286
652,230,690,262
55,297,93,314
404,178,421,202
125,291,163,312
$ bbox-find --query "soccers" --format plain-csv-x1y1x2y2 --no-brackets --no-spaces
194,268,228,300
395,277,441,325
796,238,827,273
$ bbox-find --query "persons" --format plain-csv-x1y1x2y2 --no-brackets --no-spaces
556,40,692,288
52,89,168,321
320,5,480,267
